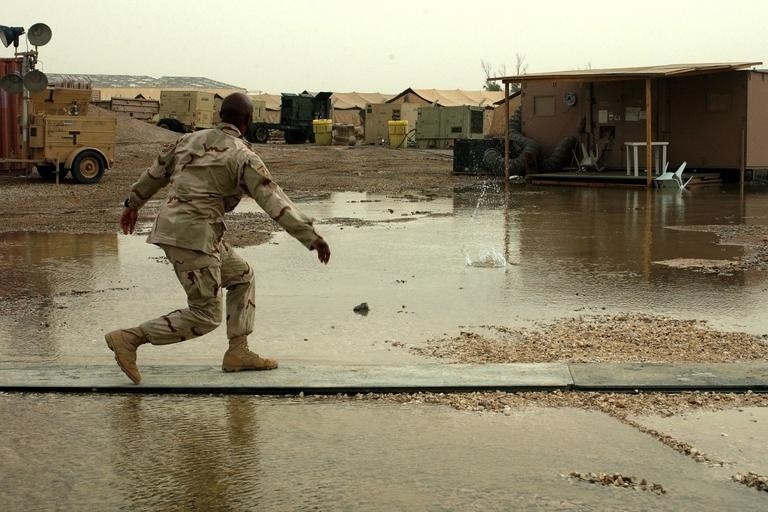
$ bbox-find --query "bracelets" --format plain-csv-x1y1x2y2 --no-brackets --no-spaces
124,198,130,208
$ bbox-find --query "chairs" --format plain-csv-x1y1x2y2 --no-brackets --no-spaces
654,160,693,191
572,143,609,172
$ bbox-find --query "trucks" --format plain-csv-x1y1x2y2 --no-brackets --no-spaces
156,90,333,144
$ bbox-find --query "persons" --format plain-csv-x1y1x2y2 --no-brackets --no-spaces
105,93,330,383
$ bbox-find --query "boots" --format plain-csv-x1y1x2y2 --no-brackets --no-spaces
105,327,147,384
222,335,278,372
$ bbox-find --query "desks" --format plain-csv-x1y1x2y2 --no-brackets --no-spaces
624,141,669,176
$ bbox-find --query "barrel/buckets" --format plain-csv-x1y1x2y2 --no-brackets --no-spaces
387,120,408,148
312,119,333,145
